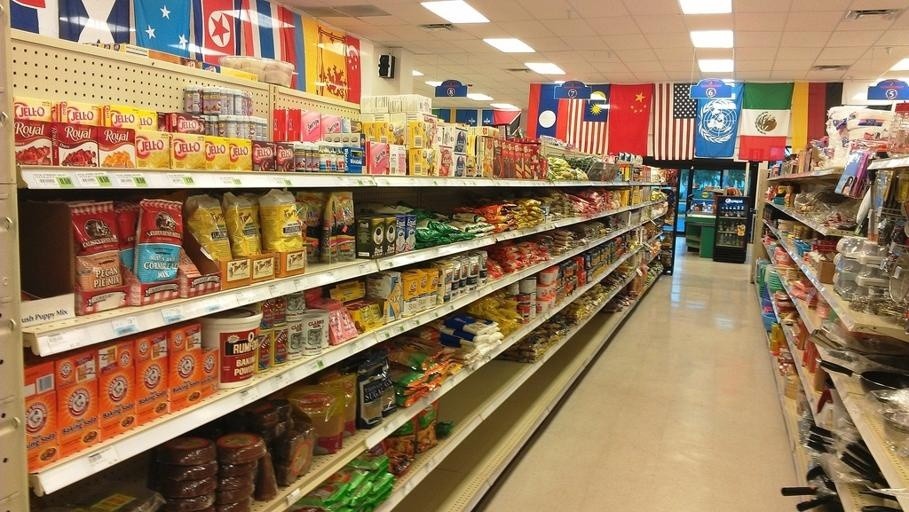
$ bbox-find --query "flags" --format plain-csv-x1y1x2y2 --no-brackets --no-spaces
526,80,844,161
10,1,360,102
